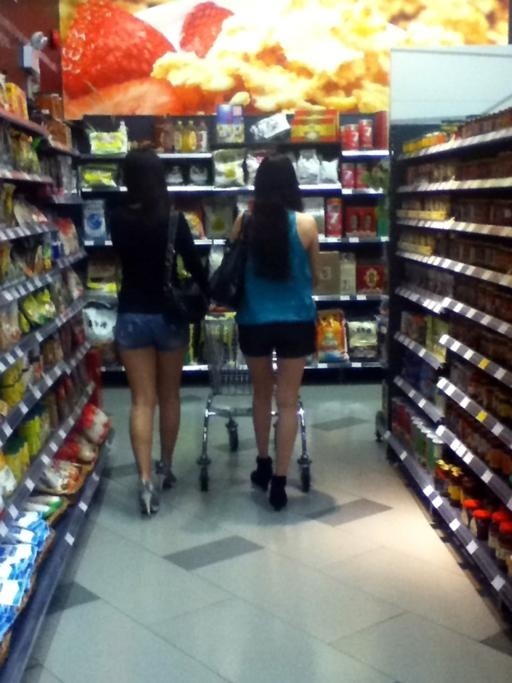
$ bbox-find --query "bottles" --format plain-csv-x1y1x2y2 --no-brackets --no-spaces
432,458,474,508
156,112,209,154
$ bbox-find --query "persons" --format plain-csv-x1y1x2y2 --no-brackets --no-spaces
228,153,322,510
104,148,214,516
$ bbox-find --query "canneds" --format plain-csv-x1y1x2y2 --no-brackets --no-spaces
435,459,512,578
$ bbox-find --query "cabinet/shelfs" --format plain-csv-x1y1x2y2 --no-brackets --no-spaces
340,145,391,370
67,114,344,374
0,102,114,682
375,114,511,630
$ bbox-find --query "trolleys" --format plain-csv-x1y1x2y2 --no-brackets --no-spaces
191,282,315,495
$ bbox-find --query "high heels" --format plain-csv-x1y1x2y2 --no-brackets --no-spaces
137,479,160,516
155,461,176,491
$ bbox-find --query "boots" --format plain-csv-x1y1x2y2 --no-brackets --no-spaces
270,475,293,510
250,456,275,492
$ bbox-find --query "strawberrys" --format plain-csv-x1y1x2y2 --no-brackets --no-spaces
180,2,234,58
60,0,178,100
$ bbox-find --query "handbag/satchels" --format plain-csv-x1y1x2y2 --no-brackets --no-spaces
210,237,244,310
162,275,209,325
